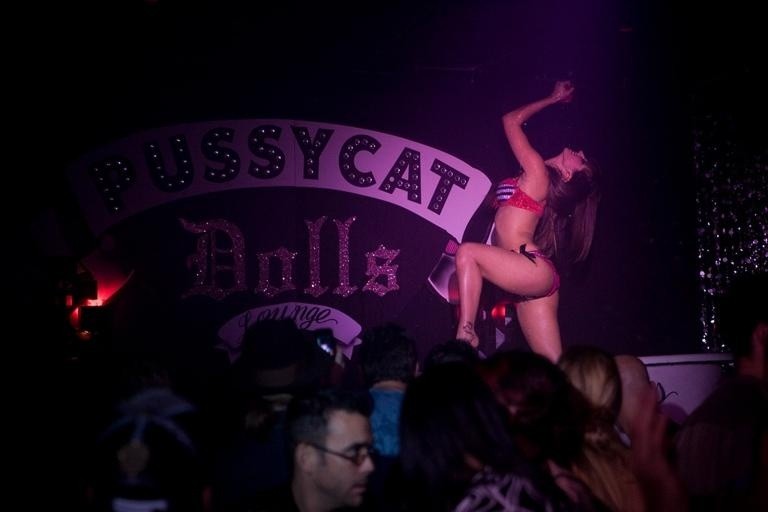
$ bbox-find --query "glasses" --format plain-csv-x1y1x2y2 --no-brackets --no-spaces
309,444,374,466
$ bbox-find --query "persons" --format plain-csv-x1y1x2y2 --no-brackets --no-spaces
0,223,768,512
452,77,602,366
